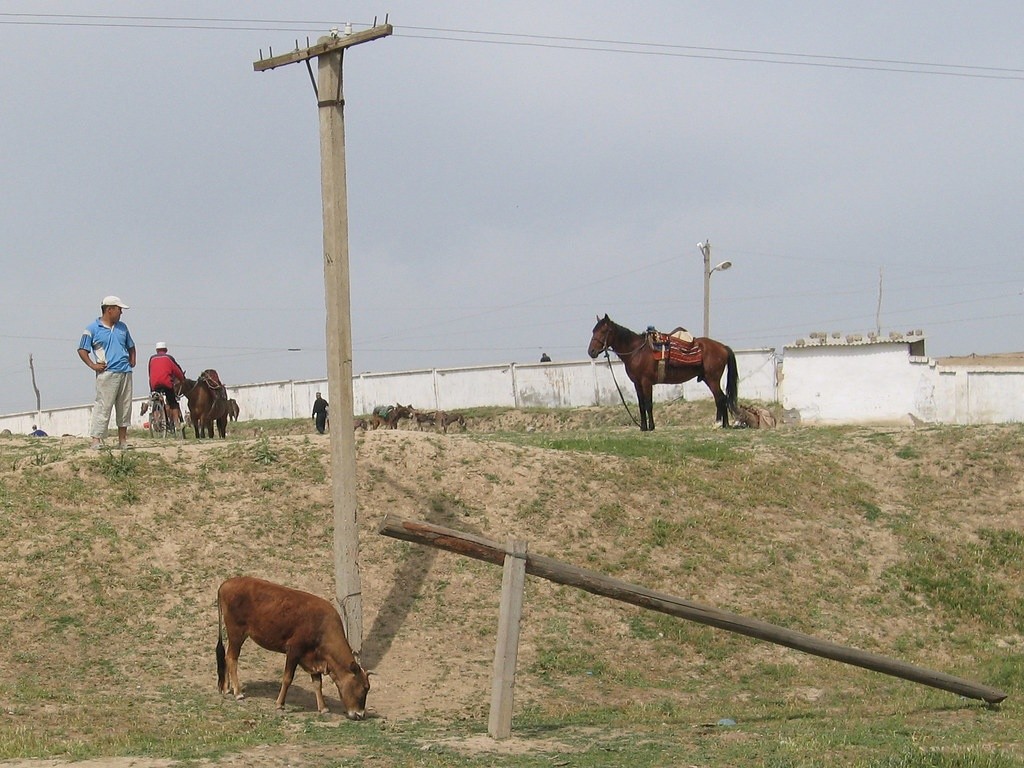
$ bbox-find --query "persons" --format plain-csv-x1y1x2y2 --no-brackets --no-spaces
77,296,137,450
147,342,187,431
540,353,551,362
311,391,328,434
31,425,48,437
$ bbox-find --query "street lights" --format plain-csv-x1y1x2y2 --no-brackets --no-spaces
697,239,732,339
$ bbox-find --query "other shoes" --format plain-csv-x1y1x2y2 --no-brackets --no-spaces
176,421,185,431
119,443,133,449
90,443,105,450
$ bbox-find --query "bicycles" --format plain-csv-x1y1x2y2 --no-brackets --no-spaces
148,382,186,441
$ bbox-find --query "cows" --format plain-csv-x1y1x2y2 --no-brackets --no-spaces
215,575,379,721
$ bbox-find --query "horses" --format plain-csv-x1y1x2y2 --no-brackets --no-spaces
174,369,240,441
587,313,739,431
353,403,466,435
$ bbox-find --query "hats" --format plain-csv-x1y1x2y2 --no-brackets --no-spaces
101,296,129,308
156,342,167,349
